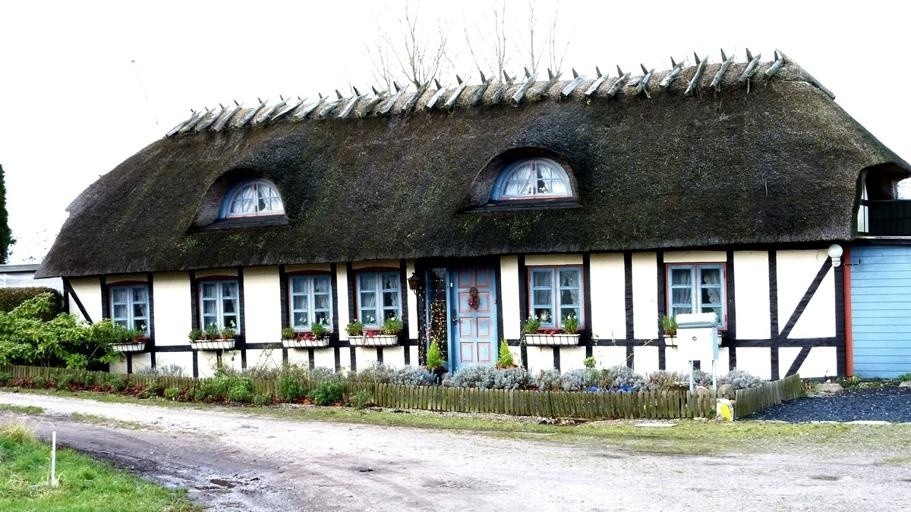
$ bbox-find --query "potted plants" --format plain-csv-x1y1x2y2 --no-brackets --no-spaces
107,326,149,353
281,322,330,348
345,314,403,347
523,313,581,346
188,324,237,350
660,314,676,346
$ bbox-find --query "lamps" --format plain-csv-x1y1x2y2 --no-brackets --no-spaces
828,244,862,268
408,272,423,296
